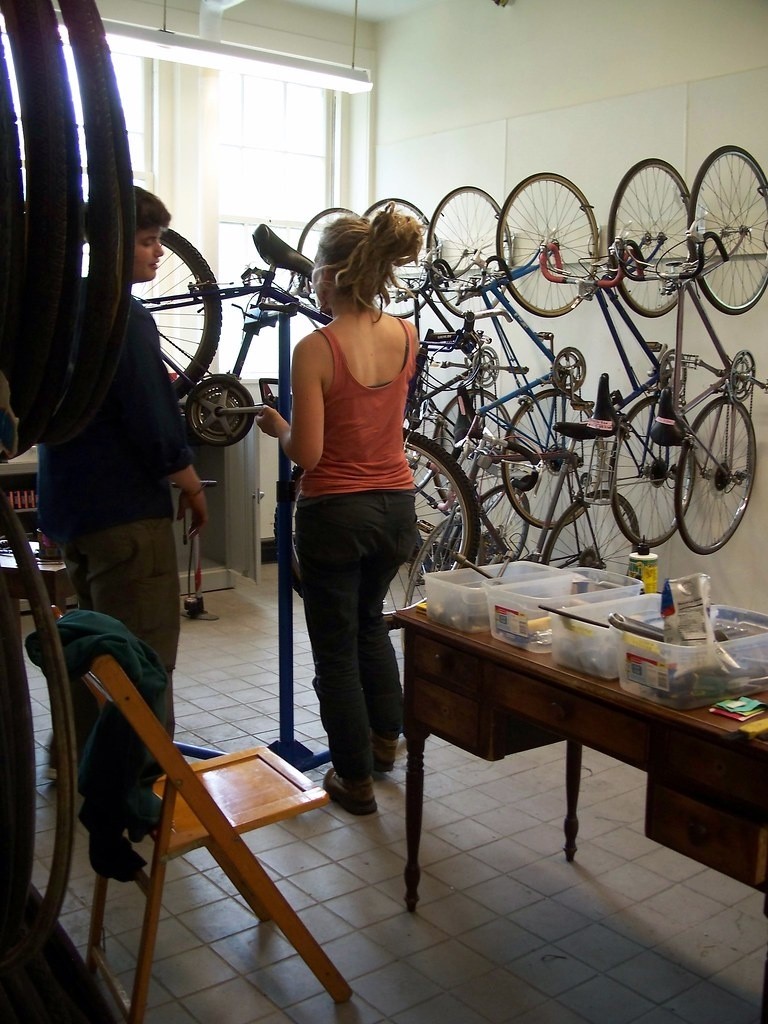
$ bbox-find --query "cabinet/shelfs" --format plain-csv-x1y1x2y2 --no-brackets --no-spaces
169,401,266,597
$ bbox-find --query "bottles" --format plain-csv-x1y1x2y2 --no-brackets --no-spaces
627,535,658,594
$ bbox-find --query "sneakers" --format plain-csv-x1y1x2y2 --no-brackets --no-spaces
371,727,400,773
322,765,378,817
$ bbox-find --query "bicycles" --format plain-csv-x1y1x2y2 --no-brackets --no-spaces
296,145,766,612
132,224,480,615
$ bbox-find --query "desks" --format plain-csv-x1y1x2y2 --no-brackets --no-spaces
390,594,767,1023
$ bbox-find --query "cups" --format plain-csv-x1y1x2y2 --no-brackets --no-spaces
37,529,61,560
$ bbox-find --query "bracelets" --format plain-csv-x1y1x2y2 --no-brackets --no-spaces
180,481,207,497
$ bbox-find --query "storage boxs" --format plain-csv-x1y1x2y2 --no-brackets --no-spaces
607,604,768,710
480,566,644,654
547,592,664,680
422,560,587,636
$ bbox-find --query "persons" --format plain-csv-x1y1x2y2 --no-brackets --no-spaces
254,201,421,817
36,184,211,782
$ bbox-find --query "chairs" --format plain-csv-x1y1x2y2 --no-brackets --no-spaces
49,603,356,1024
